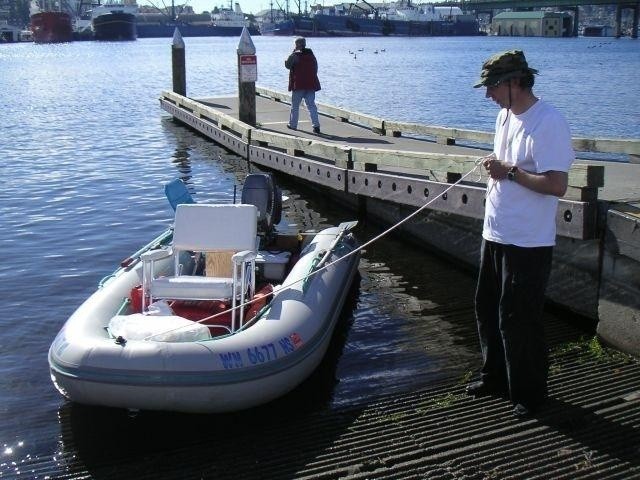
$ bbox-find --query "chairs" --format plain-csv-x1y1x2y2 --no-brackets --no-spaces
138,171,283,333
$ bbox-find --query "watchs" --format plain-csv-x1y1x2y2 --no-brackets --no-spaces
507,166,519,181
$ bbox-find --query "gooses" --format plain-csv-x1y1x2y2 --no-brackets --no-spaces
349,50,354,54
357,48,364,51
374,50,378,53
354,54,357,59
381,48,385,51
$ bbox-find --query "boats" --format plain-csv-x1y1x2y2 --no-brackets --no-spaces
133,1,483,38
46,168,363,417
29,7,74,43
71,0,102,40
91,9,138,41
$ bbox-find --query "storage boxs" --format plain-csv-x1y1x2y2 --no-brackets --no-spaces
256,249,292,281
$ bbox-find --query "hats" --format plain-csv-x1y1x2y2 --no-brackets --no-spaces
473,50,539,88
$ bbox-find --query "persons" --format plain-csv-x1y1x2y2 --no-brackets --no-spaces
284,37,322,135
464,47,577,418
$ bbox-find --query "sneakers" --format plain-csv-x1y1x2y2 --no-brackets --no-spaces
313,128,320,132
287,124,296,130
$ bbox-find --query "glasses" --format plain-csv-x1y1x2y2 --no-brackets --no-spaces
483,81,502,88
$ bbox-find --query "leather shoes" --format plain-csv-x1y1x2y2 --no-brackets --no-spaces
514,399,547,415
466,382,509,395
